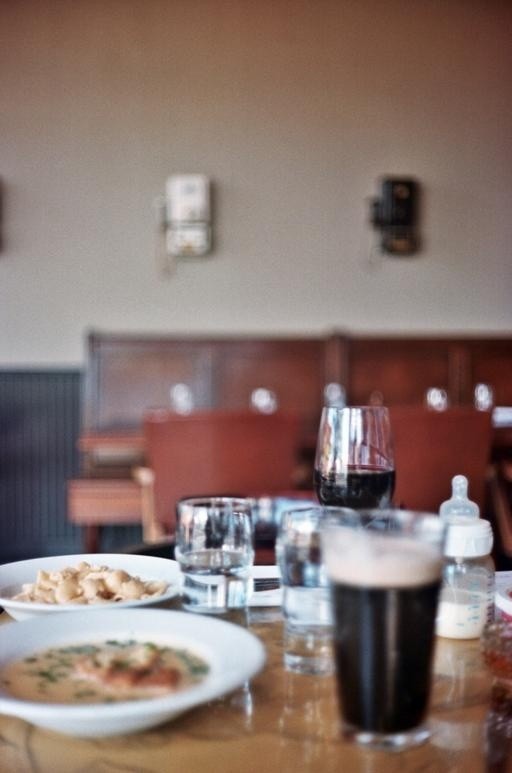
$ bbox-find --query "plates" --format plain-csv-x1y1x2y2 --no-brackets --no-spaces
0,606,267,742
0,553,184,620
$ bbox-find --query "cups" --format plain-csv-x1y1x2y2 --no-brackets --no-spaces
272,511,334,676
315,406,398,513
178,495,257,616
322,509,439,752
434,474,494,636
